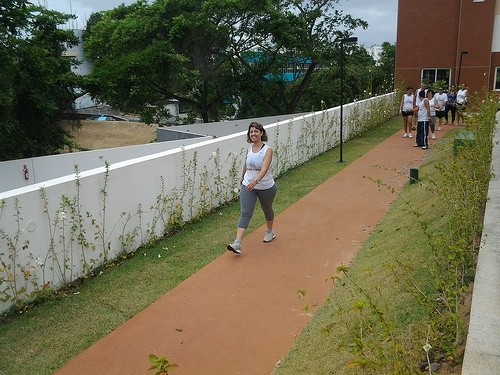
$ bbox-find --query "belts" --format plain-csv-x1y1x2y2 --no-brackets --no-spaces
246,168,261,170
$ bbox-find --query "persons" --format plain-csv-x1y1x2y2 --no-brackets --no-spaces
399,83,468,149
227,122,277,254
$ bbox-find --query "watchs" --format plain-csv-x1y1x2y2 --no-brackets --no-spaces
254,179,258,184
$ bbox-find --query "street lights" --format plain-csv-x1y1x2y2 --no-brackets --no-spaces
340,37,358,162
456,51,469,91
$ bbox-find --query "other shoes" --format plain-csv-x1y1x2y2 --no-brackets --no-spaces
413,144,421,147
421,145,428,149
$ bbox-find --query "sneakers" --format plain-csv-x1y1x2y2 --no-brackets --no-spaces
227,241,241,254
438,127,442,131
427,134,430,139
408,133,412,138
403,133,408,137
432,133,436,139
262,230,276,242
